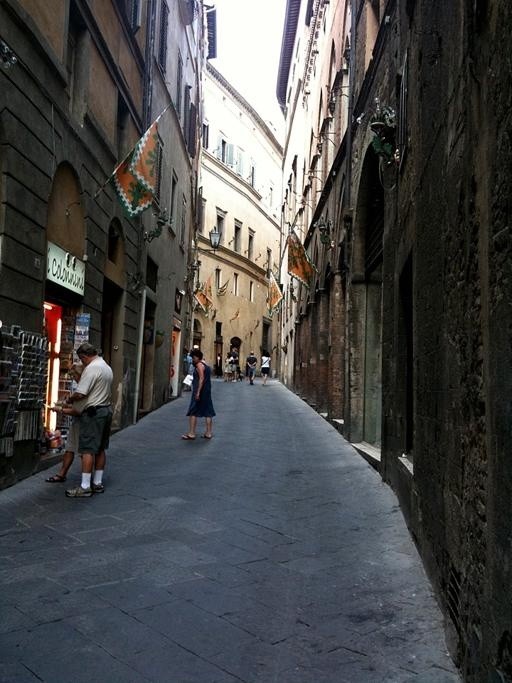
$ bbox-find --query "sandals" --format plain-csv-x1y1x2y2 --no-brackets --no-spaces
45,474,68,483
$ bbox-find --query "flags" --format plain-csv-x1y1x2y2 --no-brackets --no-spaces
267,269,284,319
286,225,319,289
112,115,160,219
194,275,215,316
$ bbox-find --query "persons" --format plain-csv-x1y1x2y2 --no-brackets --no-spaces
45,360,85,483
216,347,272,386
64,342,114,499
180,348,217,440
182,347,194,392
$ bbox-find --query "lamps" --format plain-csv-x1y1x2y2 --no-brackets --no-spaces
197,225,223,251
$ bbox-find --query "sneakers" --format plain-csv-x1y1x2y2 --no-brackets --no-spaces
64,485,93,498
90,481,106,494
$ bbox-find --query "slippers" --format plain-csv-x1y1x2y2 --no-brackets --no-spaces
200,433,212,440
181,434,196,440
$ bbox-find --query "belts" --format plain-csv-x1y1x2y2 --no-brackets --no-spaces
82,403,111,412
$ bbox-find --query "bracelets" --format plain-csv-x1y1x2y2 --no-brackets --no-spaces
57,405,63,413
